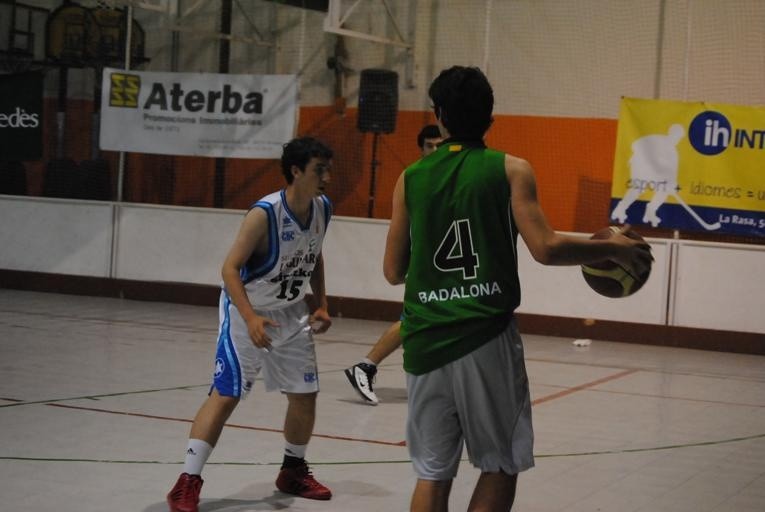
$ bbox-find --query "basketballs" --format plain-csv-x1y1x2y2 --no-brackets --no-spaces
581,224,652,298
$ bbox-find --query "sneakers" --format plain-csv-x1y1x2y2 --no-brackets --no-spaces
276,456,333,501
168,473,204,512
611,203,628,221
344,363,379,405
643,207,661,224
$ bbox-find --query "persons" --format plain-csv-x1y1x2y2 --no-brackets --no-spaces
167,137,332,512
345,127,443,405
385,64,650,512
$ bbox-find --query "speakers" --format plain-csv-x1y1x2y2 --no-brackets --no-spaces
357,68,399,134
